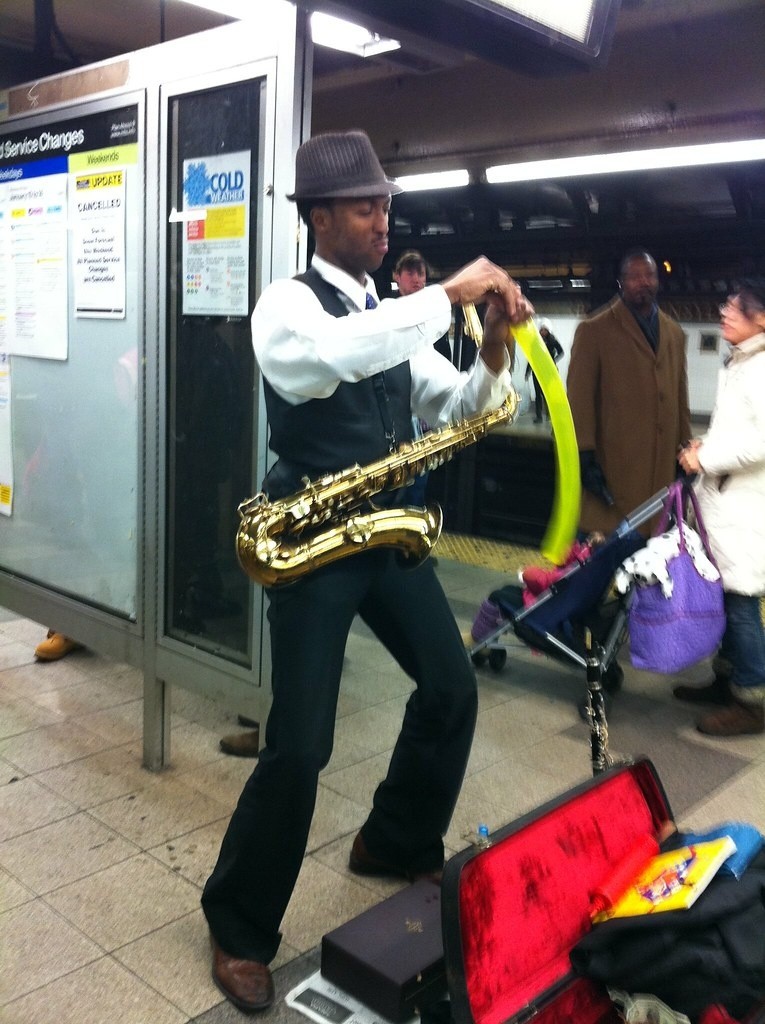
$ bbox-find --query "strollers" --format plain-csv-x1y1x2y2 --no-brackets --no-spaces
462,465,700,698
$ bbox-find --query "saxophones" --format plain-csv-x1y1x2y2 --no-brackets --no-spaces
235,383,524,589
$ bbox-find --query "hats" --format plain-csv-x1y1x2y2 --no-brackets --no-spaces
286,130,404,200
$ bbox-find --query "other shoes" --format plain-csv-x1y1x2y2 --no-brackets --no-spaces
35,630,85,660
672,658,735,707
238,713,259,729
219,730,259,755
697,686,765,737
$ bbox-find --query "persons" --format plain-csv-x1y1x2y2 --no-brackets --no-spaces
671,278,765,736
566,251,695,542
462,533,606,654
218,716,259,758
198,132,536,1014
33,629,88,664
388,252,454,508
525,324,564,423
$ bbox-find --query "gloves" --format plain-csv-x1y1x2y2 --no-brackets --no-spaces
674,460,698,486
578,449,607,498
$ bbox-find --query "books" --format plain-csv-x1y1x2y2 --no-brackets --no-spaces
595,835,737,923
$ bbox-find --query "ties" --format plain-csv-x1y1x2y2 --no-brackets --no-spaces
364,291,378,309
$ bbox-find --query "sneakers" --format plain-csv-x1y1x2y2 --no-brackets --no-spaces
64,645,66,646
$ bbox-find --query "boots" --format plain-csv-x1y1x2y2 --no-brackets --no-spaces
545,401,550,421
533,396,542,424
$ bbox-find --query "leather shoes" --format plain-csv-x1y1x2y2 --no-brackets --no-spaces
350,829,449,889
208,924,275,1011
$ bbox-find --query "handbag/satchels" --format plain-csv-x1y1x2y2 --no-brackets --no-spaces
624,482,728,678
